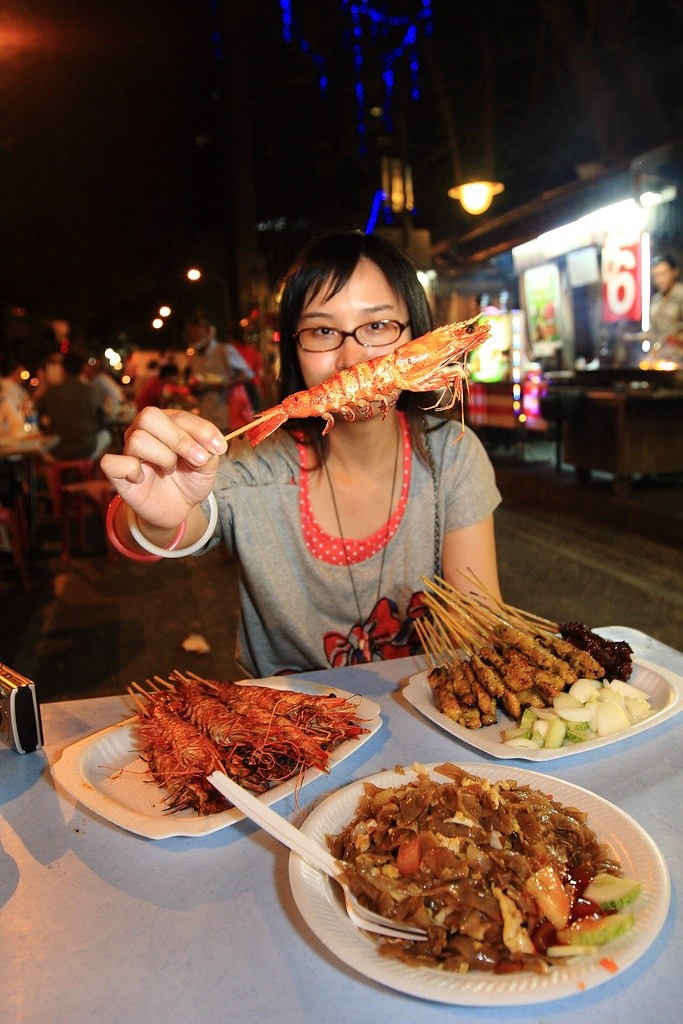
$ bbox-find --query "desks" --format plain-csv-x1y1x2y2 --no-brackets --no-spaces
0,624,683,1024
0,435,60,597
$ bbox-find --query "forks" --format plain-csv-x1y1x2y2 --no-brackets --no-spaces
209,770,428,941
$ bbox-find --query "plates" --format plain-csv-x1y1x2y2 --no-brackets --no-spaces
288,761,672,1006
49,676,382,840
402,652,683,761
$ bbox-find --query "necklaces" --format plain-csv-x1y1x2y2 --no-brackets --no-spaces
318,422,400,632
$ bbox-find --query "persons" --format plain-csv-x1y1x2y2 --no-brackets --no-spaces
629,256,683,363
99,232,501,680
0,303,280,556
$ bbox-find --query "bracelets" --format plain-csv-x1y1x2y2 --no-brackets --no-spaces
105,493,189,564
127,491,218,559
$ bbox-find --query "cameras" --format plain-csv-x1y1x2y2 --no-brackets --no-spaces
0,663,44,754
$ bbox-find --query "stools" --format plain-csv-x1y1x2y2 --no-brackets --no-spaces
0,453,116,593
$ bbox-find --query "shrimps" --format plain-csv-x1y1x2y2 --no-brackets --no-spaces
241,310,491,448
98,681,374,817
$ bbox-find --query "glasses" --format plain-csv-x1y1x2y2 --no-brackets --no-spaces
291,319,410,353
